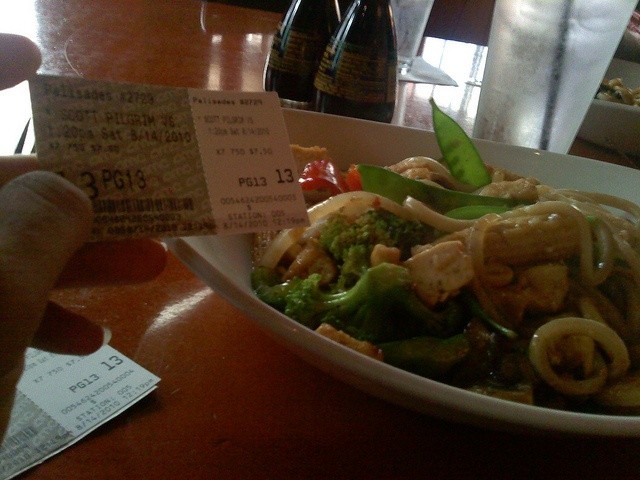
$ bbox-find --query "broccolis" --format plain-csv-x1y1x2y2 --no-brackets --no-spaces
318,210,433,265
282,261,408,339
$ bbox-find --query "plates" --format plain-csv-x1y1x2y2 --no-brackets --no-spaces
163,106,640,436
578,59,640,155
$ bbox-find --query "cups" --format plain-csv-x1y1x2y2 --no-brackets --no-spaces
471,0,640,156
391,1,434,74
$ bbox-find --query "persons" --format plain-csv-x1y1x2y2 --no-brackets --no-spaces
1,31,166,446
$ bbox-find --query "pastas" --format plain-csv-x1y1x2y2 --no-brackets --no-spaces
248,155,640,414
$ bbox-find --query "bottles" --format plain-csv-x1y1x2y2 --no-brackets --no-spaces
313,1,398,123
262,0,342,108
